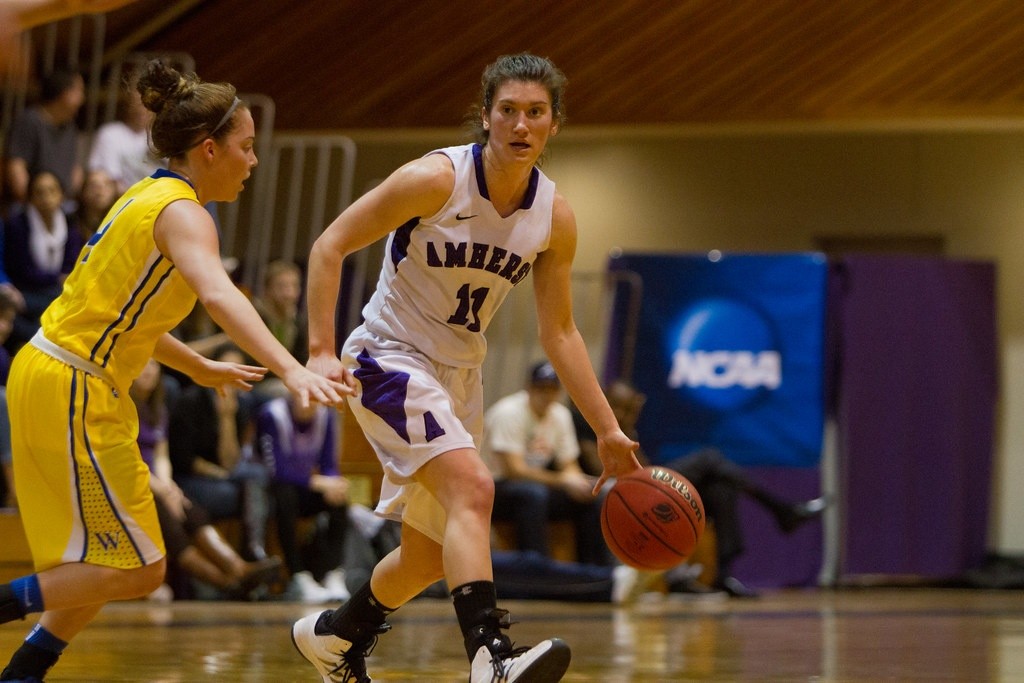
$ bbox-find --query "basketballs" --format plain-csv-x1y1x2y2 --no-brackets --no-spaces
601,465,706,573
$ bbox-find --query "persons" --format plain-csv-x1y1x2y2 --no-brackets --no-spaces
1,67,358,681
1,64,405,604
291,53,642,683
473,362,828,600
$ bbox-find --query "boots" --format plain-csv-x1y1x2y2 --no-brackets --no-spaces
178,527,283,602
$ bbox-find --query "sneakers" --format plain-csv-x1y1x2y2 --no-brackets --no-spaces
291,609,372,683
469,637,571,683
292,569,351,604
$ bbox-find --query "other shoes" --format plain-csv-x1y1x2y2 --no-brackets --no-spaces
711,577,757,599
778,492,835,537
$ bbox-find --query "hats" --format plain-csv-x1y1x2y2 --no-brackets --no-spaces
533,363,560,385
